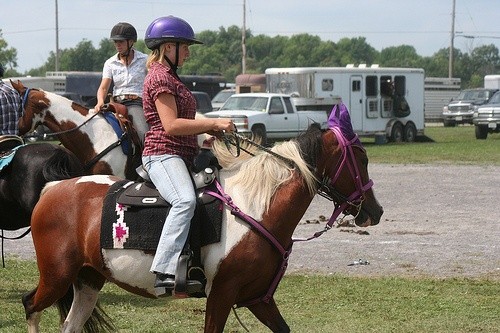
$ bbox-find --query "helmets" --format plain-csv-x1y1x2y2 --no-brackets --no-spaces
144,16,203,49
111,23,137,42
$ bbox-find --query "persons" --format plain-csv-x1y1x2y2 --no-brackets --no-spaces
96,22,148,145
142,16,236,291
0,63,23,158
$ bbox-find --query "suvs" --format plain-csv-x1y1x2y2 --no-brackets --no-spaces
472,90,500,139
442,88,500,127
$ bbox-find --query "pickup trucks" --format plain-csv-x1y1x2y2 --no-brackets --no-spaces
203,93,329,143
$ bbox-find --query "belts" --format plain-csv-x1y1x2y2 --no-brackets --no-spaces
113,95,138,100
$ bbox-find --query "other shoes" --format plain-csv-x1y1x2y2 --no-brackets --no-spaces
154,272,202,289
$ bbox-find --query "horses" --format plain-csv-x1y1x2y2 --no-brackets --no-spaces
0,143,116,332
9,78,218,175
21,104,385,333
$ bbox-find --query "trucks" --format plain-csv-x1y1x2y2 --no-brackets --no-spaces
2,70,236,115
264,62,424,146
